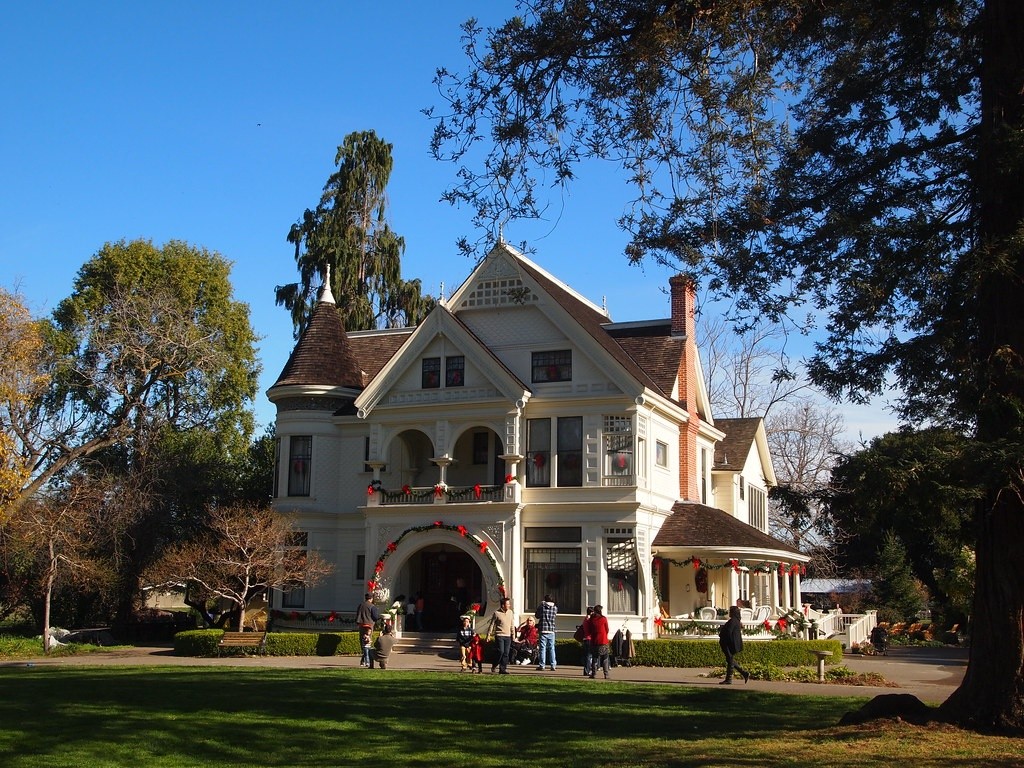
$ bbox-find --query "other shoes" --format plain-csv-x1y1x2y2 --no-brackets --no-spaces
491,668,496,674
719,681,733,685
515,660,521,665
521,658,531,665
478,671,482,674
744,672,750,684
468,665,472,669
472,669,476,674
381,663,386,669
499,670,510,674
552,668,556,671
536,667,544,671
461,668,466,671
589,676,596,678
605,675,610,679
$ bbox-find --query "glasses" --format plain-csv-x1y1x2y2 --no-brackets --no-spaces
527,620,532,622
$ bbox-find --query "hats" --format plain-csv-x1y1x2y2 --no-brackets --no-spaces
365,592,375,599
473,634,481,641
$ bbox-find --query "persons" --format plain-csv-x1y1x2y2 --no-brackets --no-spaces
354,592,424,669
449,576,539,675
871,623,888,656
717,606,750,684
535,593,558,670
573,604,610,680
836,604,843,631
736,598,745,608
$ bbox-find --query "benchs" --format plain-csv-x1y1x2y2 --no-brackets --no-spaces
217,632,266,658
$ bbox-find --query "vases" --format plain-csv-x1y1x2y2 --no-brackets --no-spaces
397,615,405,633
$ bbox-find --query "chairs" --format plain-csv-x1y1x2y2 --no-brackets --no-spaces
702,607,717,621
754,605,772,620
740,608,754,620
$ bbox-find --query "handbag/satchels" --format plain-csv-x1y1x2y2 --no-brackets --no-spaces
573,625,585,642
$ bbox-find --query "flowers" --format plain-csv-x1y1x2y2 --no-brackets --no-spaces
652,608,808,632
366,522,506,615
367,475,514,498
616,554,807,590
534,455,544,468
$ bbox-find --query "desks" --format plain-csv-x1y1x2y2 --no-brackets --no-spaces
73,627,107,645
